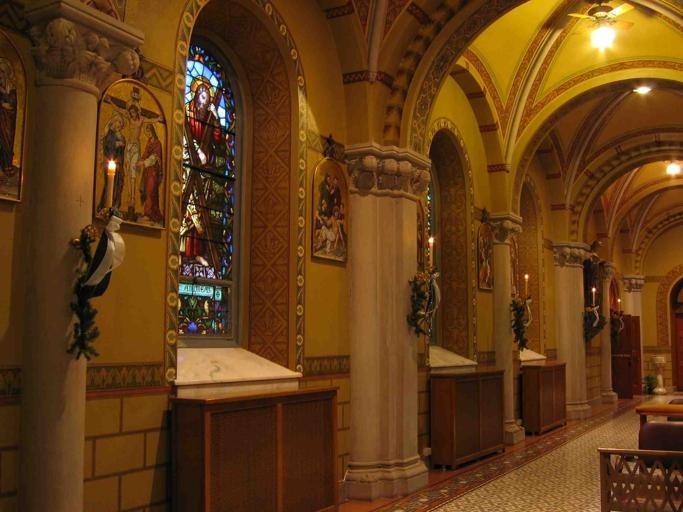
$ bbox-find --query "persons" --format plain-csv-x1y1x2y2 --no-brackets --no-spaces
478,226,493,287
313,170,346,256
179,85,225,268
99,92,165,224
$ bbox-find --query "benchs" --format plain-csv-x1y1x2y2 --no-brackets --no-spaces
594,397,682,511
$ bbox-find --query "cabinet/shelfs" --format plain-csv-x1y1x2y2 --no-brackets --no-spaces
169,384,340,511
519,359,570,438
430,362,506,471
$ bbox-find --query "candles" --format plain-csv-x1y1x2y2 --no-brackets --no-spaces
616,297,621,314
426,234,434,269
591,286,598,306
522,273,531,300
101,159,116,209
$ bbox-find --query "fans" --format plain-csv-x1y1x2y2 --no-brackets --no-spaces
564,0,636,31
